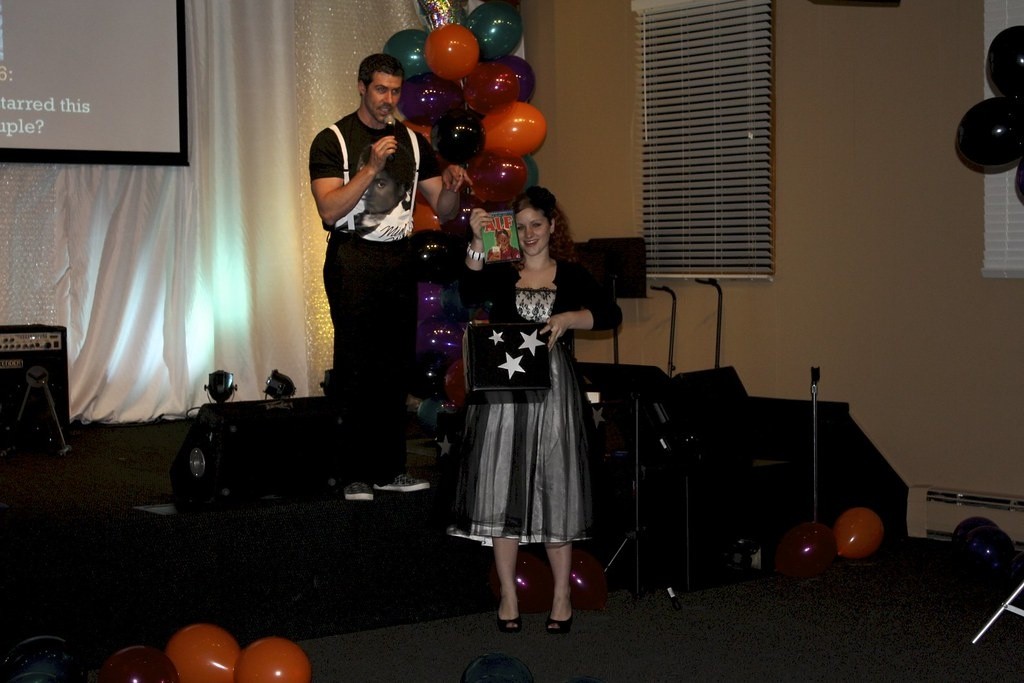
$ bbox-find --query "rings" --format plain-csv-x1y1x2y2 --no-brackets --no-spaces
459,175,464,177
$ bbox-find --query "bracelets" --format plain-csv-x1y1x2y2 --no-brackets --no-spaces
466,242,485,261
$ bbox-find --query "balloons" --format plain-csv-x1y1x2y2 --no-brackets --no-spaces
489,550,608,613
233,637,312,683
97,645,180,683
775,522,837,578
956,26,1024,207
382,0,547,443
460,653,534,683
0,636,87,683
951,516,1024,602
833,507,884,558
165,622,240,683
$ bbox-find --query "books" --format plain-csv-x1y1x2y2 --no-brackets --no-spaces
481,209,523,264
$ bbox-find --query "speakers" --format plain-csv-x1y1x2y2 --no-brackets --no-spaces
0,324,71,459
170,395,360,516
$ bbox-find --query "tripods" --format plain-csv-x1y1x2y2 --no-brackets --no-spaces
576,364,681,612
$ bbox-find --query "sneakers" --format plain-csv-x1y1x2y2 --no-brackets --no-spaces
343,481,373,501
373,473,430,492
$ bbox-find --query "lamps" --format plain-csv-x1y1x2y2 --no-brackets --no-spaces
203,369,239,403
264,368,296,401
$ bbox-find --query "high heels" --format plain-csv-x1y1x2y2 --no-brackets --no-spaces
497,608,522,634
545,608,573,634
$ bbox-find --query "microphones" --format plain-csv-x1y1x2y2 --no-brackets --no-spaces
384,113,395,161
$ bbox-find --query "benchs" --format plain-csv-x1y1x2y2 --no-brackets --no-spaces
131,487,485,648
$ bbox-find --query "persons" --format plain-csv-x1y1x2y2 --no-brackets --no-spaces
335,144,414,242
309,53,473,500
446,187,623,634
